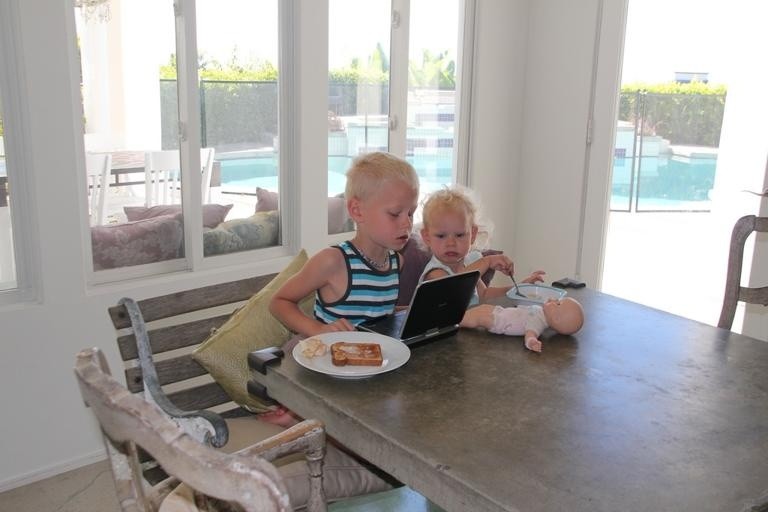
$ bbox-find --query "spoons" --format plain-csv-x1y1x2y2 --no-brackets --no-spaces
507,269,527,298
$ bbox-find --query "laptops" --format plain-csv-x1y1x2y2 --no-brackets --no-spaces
354,267,481,352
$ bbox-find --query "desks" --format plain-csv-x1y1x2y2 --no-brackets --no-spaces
247,274,766,511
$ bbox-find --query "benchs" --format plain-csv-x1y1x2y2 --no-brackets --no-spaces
97,271,398,511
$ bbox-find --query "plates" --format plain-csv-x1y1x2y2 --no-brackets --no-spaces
291,330,412,381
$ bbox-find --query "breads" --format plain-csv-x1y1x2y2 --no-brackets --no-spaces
331,342,383,366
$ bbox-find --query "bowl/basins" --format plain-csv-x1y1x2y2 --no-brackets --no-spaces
504,283,568,307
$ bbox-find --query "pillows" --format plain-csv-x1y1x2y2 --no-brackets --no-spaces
188,246,323,415
91,184,352,267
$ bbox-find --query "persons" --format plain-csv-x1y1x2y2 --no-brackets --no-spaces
268,152,419,337
411,187,547,307
455,296,585,353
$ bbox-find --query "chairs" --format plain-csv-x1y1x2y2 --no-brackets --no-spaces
88,147,214,226
74,344,331,511
